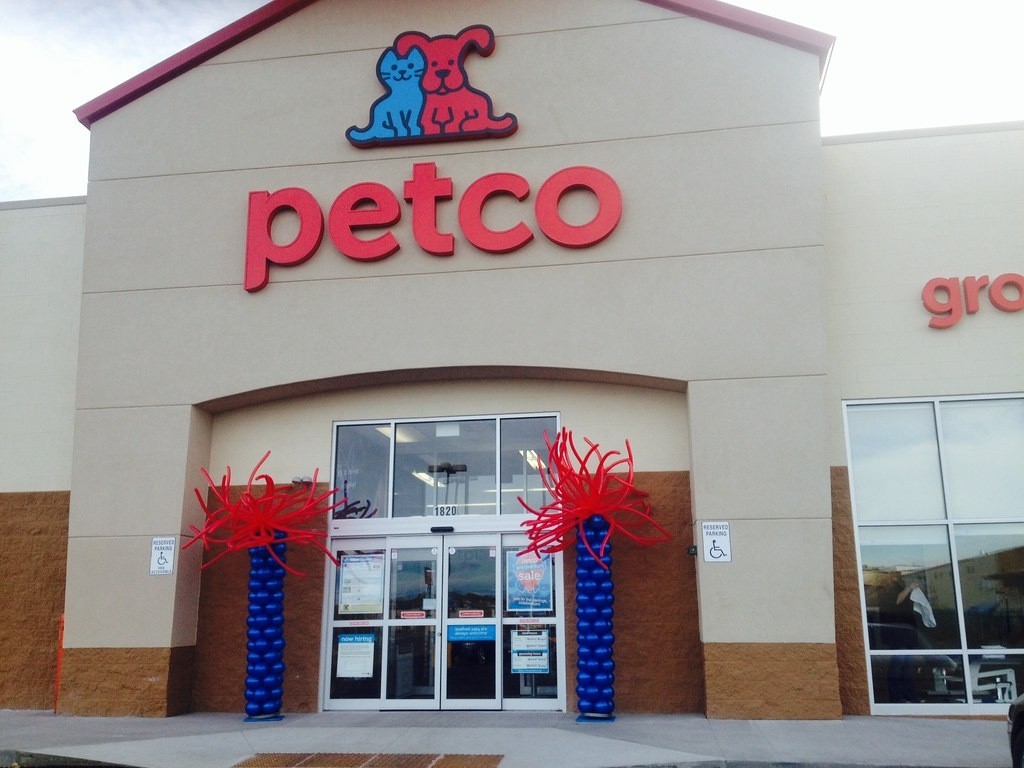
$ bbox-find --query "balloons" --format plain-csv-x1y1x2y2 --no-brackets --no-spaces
333,480,379,584
181,450,349,720
516,426,675,722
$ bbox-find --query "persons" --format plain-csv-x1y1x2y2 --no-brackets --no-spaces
878,564,936,704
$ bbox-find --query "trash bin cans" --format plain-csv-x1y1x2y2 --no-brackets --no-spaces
396,642,414,699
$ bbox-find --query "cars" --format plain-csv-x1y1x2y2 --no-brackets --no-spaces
869,622,958,695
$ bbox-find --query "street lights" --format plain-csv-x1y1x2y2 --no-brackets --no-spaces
429,462,467,510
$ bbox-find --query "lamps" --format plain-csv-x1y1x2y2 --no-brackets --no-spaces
412,466,447,488
519,450,546,471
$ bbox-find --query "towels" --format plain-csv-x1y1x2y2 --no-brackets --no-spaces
909,586,937,628
